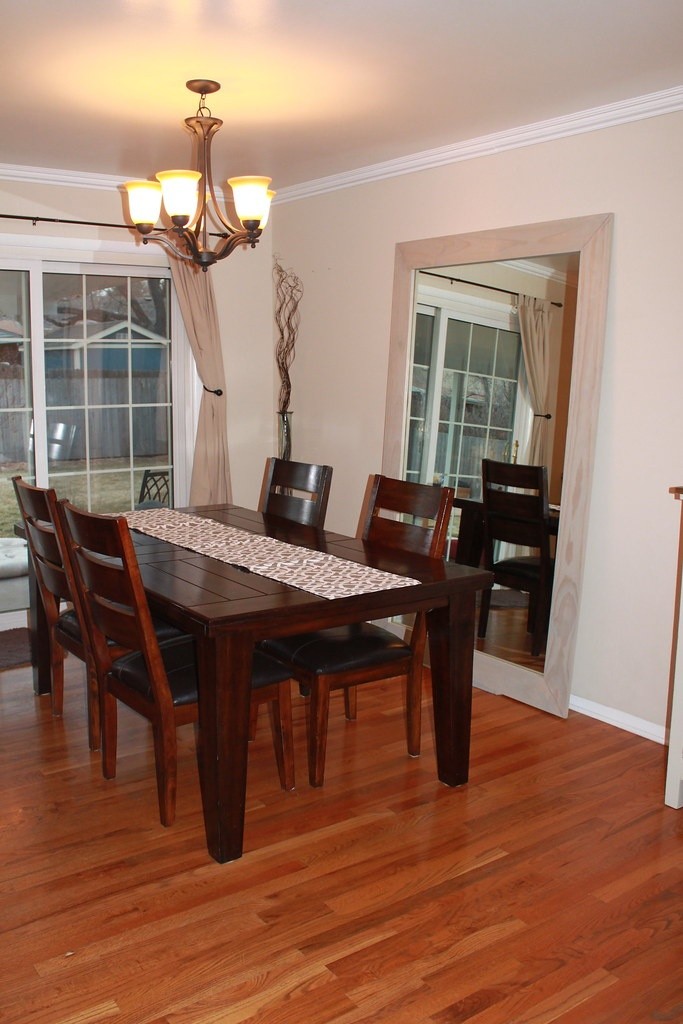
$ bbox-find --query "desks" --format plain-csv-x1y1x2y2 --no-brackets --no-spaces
78,505,495,864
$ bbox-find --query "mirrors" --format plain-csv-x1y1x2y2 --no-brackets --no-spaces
380,213,659,720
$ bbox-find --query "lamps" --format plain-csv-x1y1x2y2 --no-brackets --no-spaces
123,79,277,271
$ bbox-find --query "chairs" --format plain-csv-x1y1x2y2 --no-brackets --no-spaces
477,458,557,657
10,476,192,751
257,457,333,530
262,474,454,788
450,498,487,569
58,498,297,826
